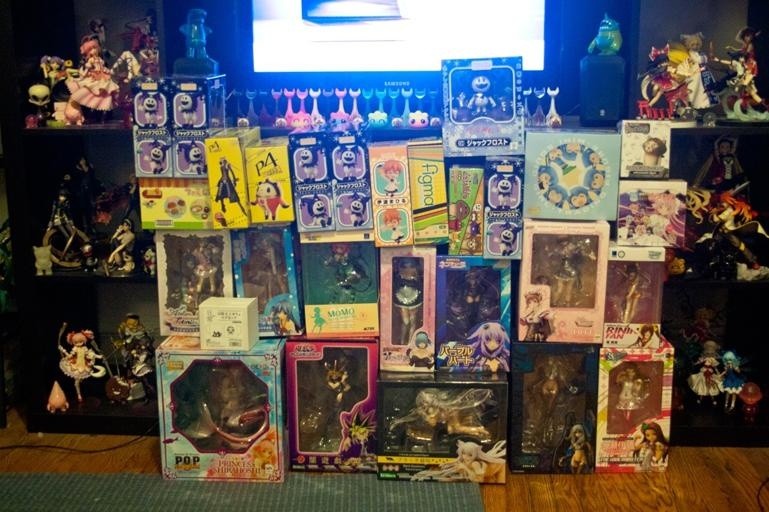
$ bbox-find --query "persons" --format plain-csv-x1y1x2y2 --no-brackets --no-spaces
25,13,764,486
25,14,763,488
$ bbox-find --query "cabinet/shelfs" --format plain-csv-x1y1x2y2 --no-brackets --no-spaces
669,122,769,448
21,120,157,435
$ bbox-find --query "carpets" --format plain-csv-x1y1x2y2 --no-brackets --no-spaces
0,471,489,510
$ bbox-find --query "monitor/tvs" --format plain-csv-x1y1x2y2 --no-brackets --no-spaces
235,0,563,91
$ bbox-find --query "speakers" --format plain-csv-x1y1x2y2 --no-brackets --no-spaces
579,55,625,126
173,59,218,77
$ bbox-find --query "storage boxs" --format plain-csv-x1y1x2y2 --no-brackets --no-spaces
134,53,689,484
